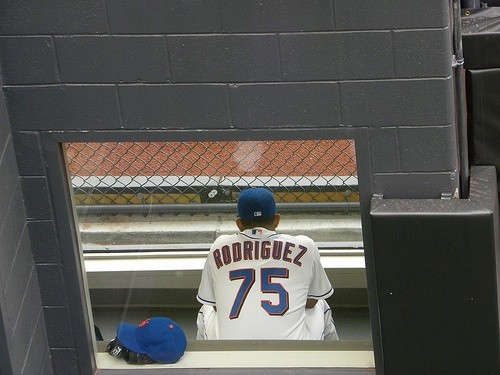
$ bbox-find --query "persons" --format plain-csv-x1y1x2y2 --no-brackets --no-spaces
194,187,340,342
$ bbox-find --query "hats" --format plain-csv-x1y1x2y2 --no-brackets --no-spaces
117,316,187,365
237,186,277,222
